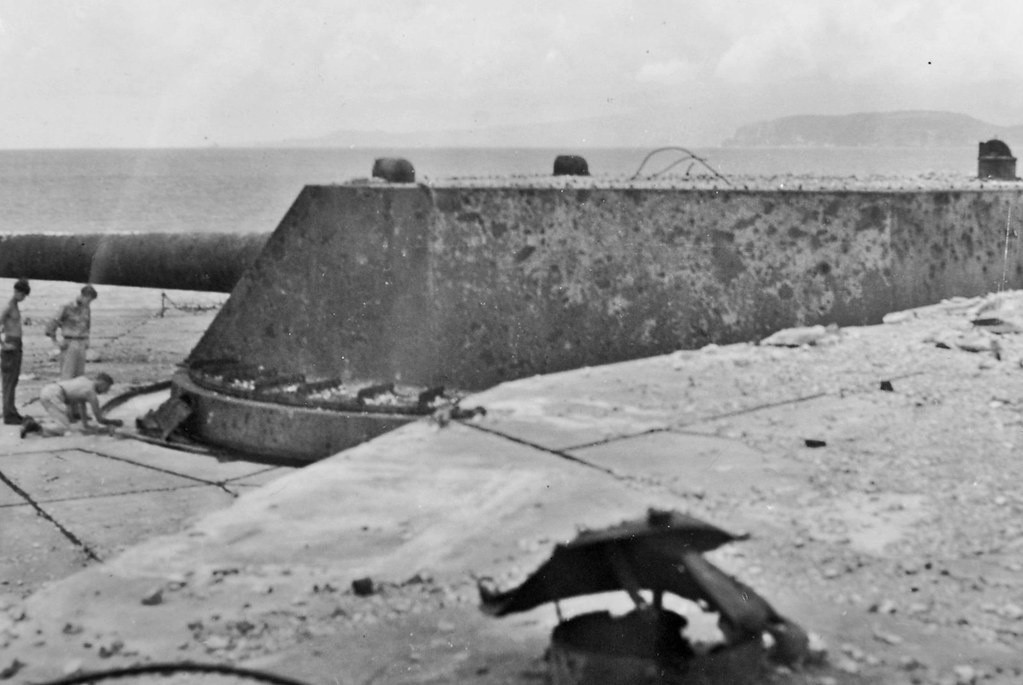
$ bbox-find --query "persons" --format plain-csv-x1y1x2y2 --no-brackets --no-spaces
0,281,30,425
45,285,97,424
20,372,123,440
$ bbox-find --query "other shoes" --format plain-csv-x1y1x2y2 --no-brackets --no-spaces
4,414,23,424
21,416,38,439
